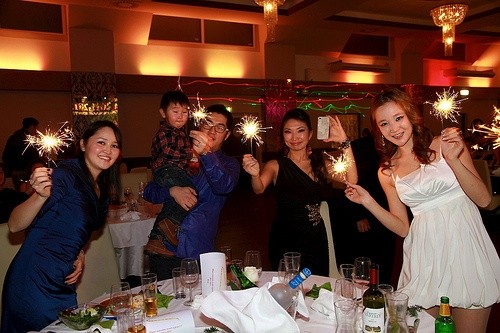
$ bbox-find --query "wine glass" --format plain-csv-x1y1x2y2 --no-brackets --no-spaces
180,258,200,306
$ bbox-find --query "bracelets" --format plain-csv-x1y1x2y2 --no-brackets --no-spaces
340,137,350,148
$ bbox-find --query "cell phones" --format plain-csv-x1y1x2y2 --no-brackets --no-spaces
317,116,329,140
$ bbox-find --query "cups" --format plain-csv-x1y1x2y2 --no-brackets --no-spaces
278,252,305,320
172,267,187,299
109,282,146,333
219,245,262,286
124,186,146,212
141,273,157,318
334,255,409,333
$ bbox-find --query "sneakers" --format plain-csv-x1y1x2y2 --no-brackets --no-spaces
144,239,175,260
156,218,179,245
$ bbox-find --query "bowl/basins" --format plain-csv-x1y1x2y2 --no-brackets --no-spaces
57,302,108,331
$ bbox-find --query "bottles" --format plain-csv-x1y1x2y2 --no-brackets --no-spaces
268,267,311,311
362,263,387,333
435,296,456,333
230,263,258,289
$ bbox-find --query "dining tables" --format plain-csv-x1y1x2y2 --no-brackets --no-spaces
40,269,438,333
107,213,157,279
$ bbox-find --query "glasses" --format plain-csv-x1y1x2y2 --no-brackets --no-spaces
202,120,228,134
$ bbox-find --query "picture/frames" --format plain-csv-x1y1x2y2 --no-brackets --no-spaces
331,113,361,142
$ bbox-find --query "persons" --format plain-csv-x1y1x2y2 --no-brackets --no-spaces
241,108,358,278
0,121,123,333
349,130,414,291
343,88,500,333
142,90,241,279
2,117,45,180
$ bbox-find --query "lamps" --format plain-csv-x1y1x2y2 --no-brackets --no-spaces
430,4,468,56
255,0,285,26
